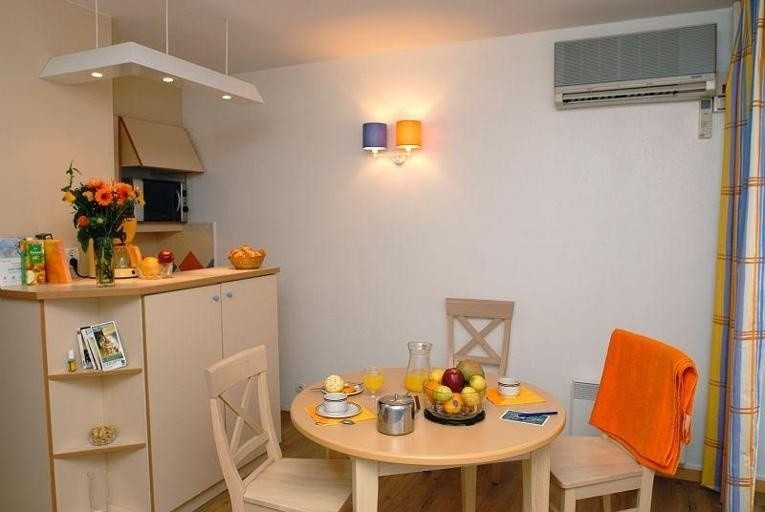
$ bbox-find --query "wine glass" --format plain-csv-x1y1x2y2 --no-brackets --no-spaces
362,365,386,408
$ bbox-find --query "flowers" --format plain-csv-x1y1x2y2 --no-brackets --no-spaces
56,159,144,253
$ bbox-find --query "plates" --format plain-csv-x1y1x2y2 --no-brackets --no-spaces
322,381,364,395
315,401,362,418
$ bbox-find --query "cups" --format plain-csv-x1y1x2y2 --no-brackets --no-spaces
323,392,348,413
497,378,521,397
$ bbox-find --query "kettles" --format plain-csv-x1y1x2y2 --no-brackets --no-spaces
113,243,138,278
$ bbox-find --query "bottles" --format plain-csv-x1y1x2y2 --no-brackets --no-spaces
68,349,77,372
157,248,175,279
404,341,433,392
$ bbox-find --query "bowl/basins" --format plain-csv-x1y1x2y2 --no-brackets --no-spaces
228,255,266,269
422,380,486,419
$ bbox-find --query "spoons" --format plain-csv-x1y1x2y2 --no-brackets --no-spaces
316,419,354,425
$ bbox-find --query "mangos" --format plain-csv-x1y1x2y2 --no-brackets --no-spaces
428,367,445,383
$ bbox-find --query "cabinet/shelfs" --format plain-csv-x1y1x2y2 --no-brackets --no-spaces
142,273,281,512
0,289,155,512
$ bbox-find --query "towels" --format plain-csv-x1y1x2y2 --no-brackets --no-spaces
588,329,699,475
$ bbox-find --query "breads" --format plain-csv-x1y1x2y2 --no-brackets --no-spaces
324,374,344,393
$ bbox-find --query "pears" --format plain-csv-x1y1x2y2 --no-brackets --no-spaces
432,385,452,401
469,376,486,393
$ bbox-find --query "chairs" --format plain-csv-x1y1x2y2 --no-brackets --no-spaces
550,329,684,512
205,345,352,512
446,298,514,484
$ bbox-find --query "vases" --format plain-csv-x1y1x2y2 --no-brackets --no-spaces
93,236,116,286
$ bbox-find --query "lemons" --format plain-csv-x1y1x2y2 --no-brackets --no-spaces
138,257,163,277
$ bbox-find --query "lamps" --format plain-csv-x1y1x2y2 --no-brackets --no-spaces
363,120,423,165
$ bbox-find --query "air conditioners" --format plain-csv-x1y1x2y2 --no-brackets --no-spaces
553,21,717,110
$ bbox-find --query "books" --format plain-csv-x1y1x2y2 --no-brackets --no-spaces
76,319,128,374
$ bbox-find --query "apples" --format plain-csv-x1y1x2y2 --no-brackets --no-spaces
158,249,176,263
442,368,464,393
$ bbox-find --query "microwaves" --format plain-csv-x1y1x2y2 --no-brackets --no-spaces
120,176,190,223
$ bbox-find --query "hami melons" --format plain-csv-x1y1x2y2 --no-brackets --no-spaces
455,359,485,385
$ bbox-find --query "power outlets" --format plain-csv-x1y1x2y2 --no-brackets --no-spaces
65,247,80,268
